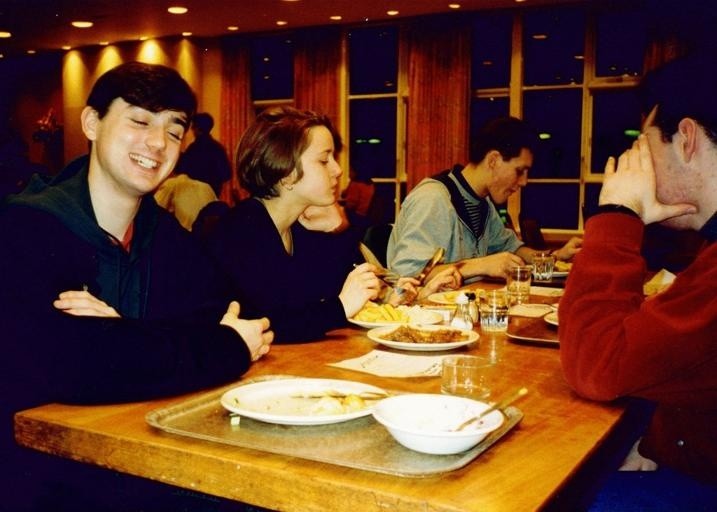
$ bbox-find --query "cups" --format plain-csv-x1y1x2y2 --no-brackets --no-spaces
505,264,533,305
438,355,497,402
479,291,512,334
531,251,554,282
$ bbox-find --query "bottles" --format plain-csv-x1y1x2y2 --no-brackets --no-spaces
453,294,475,330
465,293,478,322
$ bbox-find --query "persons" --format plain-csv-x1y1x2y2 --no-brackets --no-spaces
173,113,231,196
340,157,375,214
385,113,583,286
207,104,464,345
557,57,717,511
1,61,277,512
152,152,218,233
33,107,64,173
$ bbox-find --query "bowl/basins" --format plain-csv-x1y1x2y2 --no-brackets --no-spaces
368,395,508,458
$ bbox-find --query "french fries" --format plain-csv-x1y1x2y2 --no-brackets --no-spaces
354,301,409,325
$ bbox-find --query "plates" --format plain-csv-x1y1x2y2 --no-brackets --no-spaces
531,270,569,278
221,379,386,427
349,311,451,330
426,291,467,304
365,325,481,352
541,311,559,327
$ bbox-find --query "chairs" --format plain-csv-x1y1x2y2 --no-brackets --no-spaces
517,211,551,253
